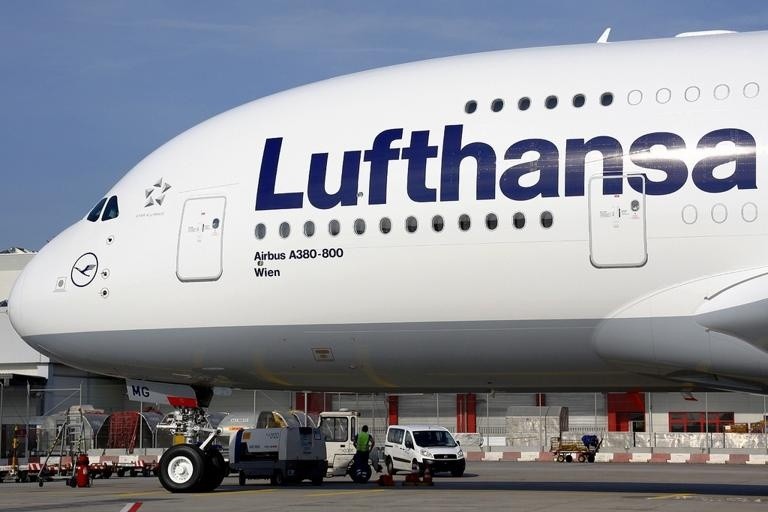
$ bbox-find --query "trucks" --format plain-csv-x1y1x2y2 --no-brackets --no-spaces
272,407,361,478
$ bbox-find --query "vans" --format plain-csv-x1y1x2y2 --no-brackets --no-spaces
382,423,467,479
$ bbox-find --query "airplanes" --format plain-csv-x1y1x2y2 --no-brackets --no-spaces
7,28,767,397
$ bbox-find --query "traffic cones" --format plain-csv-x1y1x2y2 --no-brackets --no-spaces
418,459,436,486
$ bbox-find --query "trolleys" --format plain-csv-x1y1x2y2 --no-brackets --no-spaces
543,434,608,464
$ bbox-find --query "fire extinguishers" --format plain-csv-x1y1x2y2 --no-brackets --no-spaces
75,453,91,487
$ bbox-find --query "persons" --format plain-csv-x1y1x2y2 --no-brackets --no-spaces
352,426,376,484
